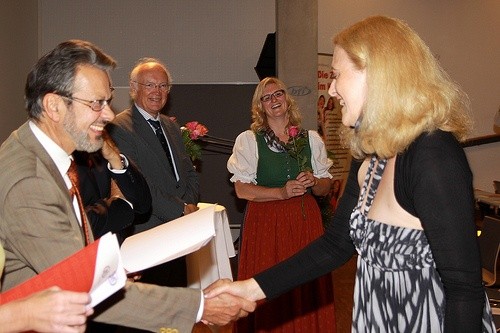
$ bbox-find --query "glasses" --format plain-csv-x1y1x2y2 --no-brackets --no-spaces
133,79,172,92
259,89,286,102
53,88,114,111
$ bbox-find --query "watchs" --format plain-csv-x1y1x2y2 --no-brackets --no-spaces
110,156,125,170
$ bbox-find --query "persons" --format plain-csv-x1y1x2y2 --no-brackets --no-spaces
71,88,152,248
0,40,257,333
227,77,336,333
0,285,94,333
200,16,496,333
104,57,200,288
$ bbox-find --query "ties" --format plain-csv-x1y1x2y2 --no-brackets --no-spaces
147,118,177,182
66,159,90,246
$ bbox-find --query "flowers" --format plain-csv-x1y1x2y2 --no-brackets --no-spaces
182,122,207,161
478,215,500,333
288,126,311,220
